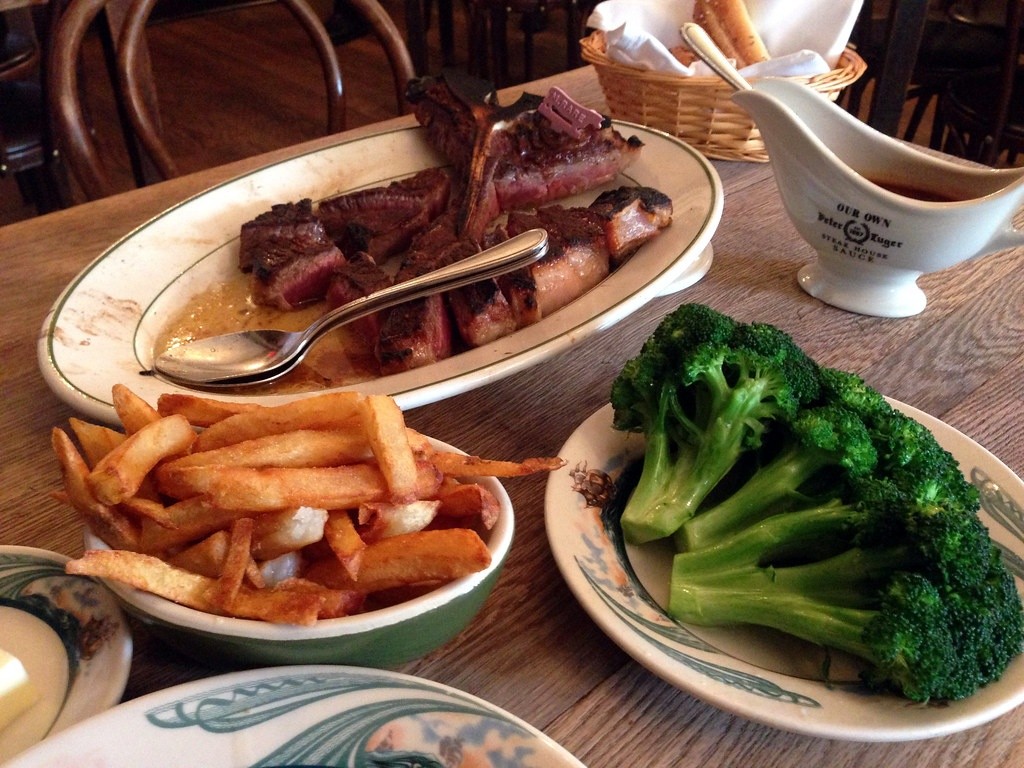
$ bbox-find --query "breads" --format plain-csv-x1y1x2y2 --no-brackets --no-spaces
666,1,770,71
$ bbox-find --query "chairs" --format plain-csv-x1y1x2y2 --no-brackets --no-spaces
48,0,416,203
833,0,1024,170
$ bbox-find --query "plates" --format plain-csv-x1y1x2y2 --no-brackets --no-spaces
0,544,132,768
0,665,588,768
36,116,724,427
544,394,1024,741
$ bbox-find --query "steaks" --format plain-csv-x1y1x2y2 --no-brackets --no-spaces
237,73,675,375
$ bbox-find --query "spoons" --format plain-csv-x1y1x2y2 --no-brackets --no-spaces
153,224,549,388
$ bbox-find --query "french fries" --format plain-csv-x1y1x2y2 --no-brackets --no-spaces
52,384,566,623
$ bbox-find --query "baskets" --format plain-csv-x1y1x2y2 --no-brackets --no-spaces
580,30,866,163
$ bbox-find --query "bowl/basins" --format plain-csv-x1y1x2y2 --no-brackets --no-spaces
85,433,514,669
735,78,1023,317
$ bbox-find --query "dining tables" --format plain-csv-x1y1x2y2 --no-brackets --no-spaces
0,68,1024,768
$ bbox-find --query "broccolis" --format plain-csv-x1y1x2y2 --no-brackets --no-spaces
609,303,1024,702
